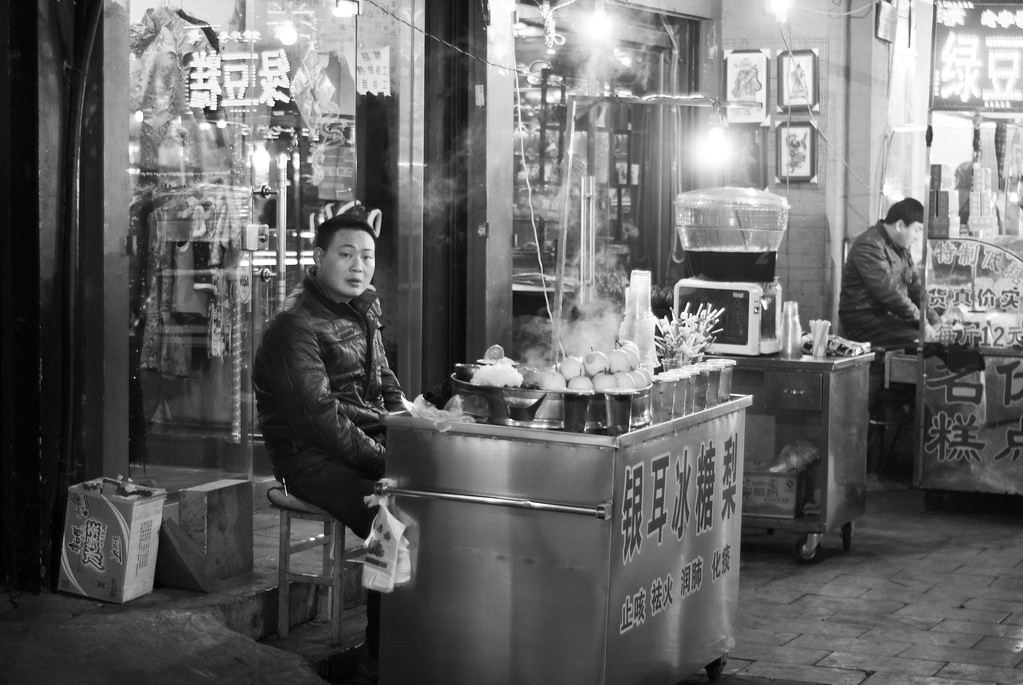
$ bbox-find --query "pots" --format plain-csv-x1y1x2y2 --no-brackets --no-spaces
452,371,653,430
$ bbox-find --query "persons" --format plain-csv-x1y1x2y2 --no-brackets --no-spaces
251,212,413,685
838,197,958,423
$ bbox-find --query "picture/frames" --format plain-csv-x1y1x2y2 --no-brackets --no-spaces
722,50,772,128
774,46,820,112
774,120,819,183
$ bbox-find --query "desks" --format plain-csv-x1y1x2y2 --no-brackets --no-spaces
702,353,877,564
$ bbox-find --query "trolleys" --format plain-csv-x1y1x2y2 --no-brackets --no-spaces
361,391,737,684
672,346,872,560
879,237,1023,495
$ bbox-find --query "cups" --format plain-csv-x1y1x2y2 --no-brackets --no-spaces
810,324,831,357
778,301,804,360
603,271,738,437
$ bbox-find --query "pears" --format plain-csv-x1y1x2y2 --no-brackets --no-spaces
537,338,652,392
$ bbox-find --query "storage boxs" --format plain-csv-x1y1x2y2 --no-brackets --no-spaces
56,476,167,605
740,470,808,520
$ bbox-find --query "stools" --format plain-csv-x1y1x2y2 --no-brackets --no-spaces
264,489,371,650
869,416,910,468
